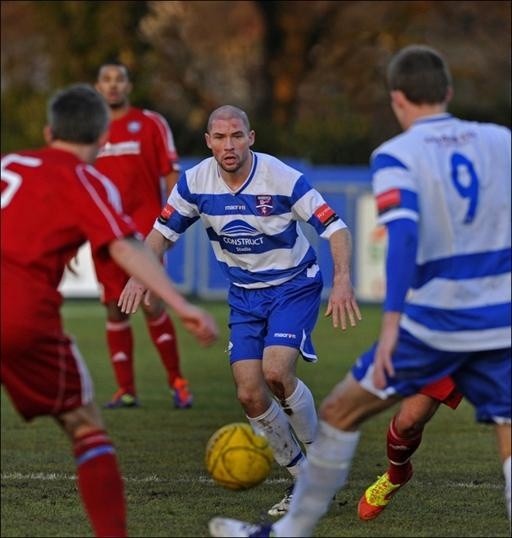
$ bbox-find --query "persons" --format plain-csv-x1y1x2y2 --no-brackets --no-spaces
209,45,512,538
117,101,366,518
358,376,463,521
1,81,219,538
88,56,202,411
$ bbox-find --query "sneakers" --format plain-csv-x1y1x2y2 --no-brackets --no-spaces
356,466,416,525
169,379,194,410
101,391,140,411
266,483,295,519
205,514,283,537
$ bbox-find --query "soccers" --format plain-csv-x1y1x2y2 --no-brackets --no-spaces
205,423,274,491
